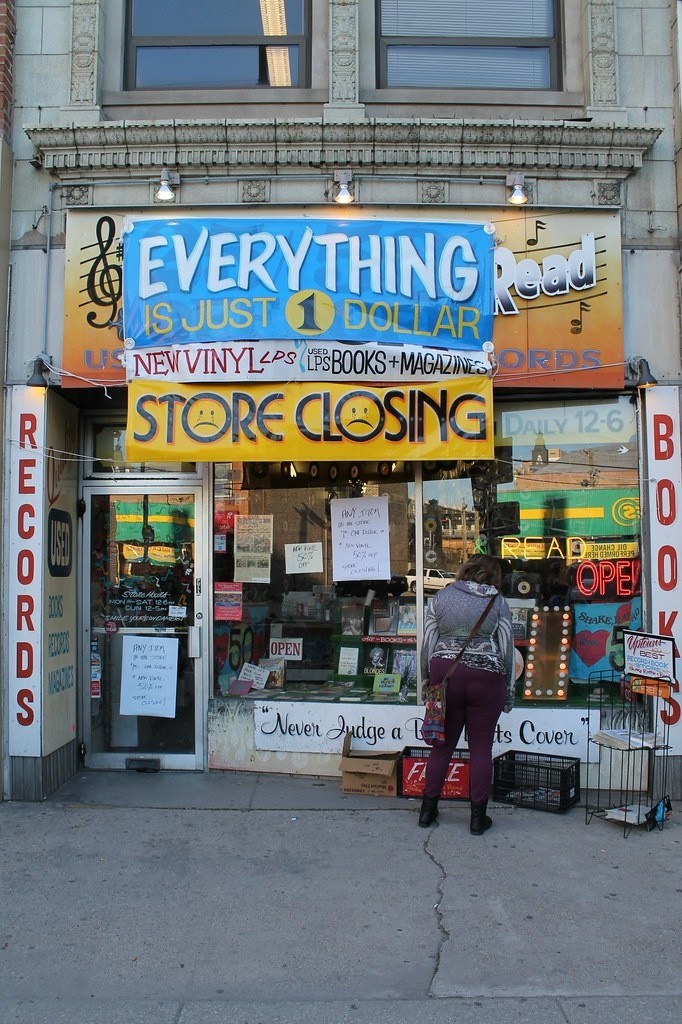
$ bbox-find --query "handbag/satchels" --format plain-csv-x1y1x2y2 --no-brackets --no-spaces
419,682,448,747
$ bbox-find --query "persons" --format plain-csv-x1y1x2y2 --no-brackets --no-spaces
417,553,516,835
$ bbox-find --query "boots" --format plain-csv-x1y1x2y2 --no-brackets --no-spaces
419,789,442,827
470,797,492,835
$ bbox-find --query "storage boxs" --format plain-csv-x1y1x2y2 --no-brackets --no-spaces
338,731,401,798
398,745,471,801
492,749,582,814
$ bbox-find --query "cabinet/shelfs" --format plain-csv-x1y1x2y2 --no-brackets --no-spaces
584,630,675,839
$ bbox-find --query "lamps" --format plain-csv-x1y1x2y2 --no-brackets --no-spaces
631,354,660,391
504,174,530,205
151,167,182,200
22,356,52,401
330,170,355,205
